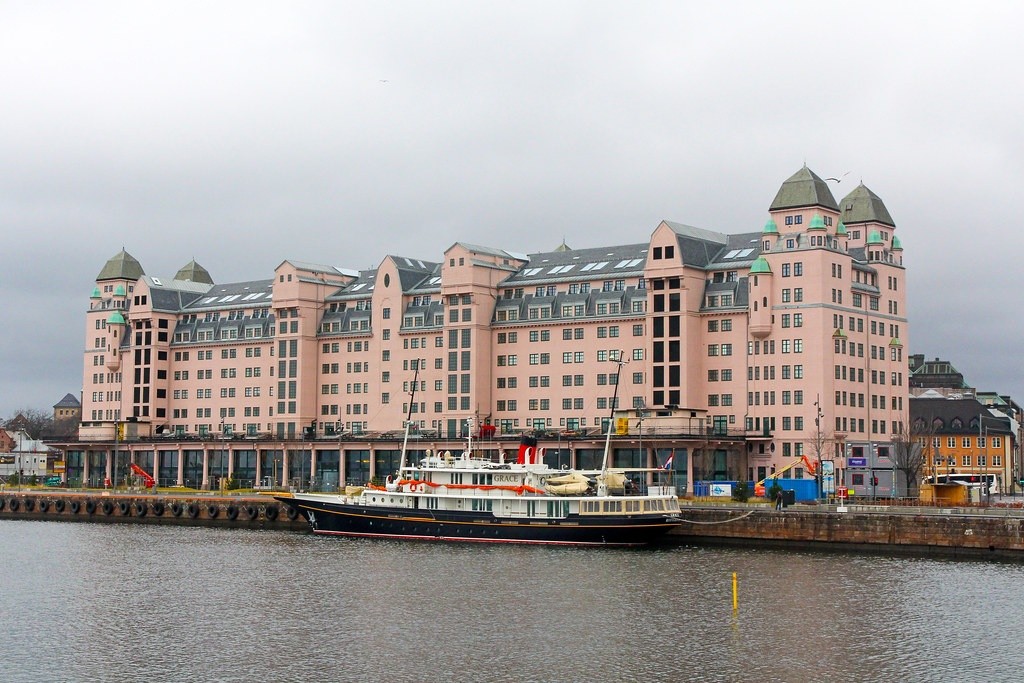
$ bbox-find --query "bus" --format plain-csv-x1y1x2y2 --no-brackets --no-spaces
921,473,1000,497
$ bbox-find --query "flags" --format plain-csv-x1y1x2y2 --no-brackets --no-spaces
665,454,673,469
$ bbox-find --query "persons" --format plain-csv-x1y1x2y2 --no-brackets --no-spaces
775,491,781,511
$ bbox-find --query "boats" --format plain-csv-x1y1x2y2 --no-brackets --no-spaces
271,345,685,549
542,471,632,497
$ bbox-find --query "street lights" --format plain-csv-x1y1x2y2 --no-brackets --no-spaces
637,398,646,493
812,393,825,510
17,420,26,491
554,429,561,469
113,405,121,490
220,415,225,497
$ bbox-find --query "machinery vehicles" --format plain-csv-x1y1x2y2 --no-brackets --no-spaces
754,453,818,498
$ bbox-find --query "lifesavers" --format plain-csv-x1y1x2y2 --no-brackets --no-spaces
247,505,258,519
287,506,299,520
172,504,183,516
410,484,417,492
10,499,19,511
0,498,5,510
188,504,199,517
135,502,147,517
153,503,164,516
208,504,219,518
540,476,546,486
39,500,49,512
70,501,80,513
266,506,278,520
103,502,113,514
86,500,96,514
25,499,35,511
119,501,130,515
226,505,239,520
55,500,65,512
